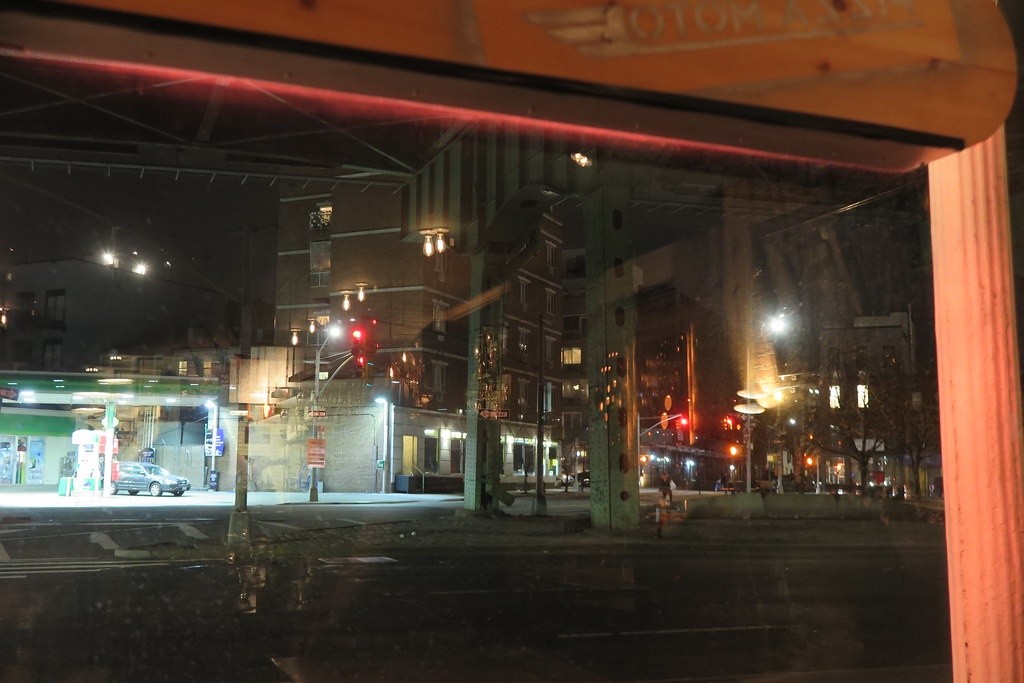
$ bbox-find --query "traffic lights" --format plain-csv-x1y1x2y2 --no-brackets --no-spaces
725,415,734,430
680,418,689,426
350,325,368,366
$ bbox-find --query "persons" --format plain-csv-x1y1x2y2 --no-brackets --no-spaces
658,471,672,509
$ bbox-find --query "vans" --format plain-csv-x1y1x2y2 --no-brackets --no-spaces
110,462,191,497
